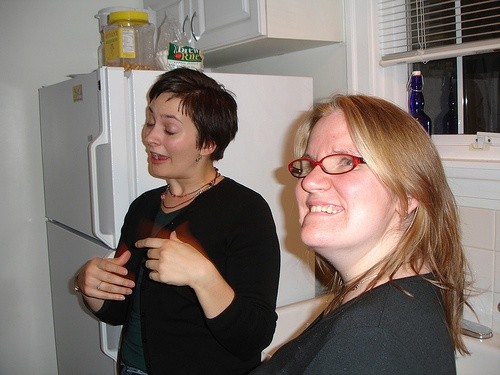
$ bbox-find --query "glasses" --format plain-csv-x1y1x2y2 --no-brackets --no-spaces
289,153,367,179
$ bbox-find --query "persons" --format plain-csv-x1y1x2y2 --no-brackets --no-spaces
72,67,280,375
248,94,479,375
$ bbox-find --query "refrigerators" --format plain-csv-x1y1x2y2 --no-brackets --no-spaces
38,67,314,374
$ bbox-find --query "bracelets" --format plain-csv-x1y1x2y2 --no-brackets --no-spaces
97,280,102,290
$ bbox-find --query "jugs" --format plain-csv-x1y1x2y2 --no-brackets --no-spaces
94,7,157,68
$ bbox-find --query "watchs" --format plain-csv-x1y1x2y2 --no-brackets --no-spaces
74,276,81,293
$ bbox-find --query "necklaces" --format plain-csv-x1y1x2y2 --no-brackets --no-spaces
351,275,378,292
160,167,220,209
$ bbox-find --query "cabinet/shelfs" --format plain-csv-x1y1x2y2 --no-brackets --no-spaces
144,0,345,68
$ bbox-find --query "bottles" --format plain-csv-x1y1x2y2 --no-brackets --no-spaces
409,71,431,138
441,74,459,135
